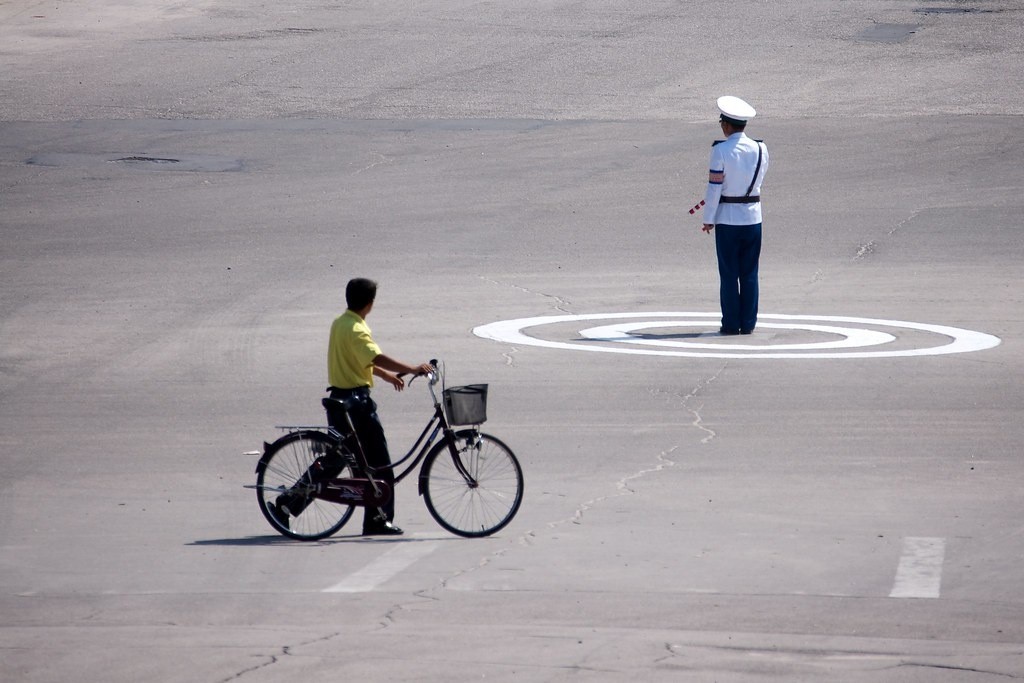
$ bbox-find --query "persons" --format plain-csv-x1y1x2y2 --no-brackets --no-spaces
702,95,769,336
264,277,434,535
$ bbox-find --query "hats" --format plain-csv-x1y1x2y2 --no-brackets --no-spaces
716,95,757,123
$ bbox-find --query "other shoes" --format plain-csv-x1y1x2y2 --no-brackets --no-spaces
720,327,752,334
362,521,404,535
263,500,291,536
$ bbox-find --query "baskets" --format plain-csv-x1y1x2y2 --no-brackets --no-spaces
443,384,488,426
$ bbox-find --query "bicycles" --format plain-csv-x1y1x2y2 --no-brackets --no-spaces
244,357,524,540
